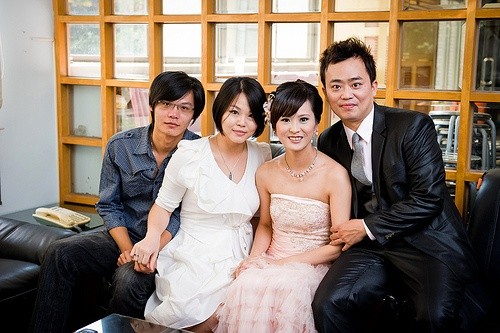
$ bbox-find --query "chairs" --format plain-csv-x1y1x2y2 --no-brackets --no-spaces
423,168,500,333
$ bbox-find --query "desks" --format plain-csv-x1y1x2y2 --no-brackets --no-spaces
72,313,195,333
0,202,104,233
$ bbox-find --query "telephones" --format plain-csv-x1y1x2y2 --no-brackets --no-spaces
32,206,90,228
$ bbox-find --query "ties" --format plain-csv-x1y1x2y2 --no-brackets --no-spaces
351,134,371,186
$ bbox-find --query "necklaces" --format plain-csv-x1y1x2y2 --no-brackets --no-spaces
216,134,246,180
285,148,318,182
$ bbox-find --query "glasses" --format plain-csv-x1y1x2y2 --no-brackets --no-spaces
157,100,195,113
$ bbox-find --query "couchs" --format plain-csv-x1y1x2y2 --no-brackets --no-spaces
0,216,76,333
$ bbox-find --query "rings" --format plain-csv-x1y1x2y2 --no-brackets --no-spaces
134,254,139,257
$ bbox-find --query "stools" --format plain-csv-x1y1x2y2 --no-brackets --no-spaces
429,111,496,212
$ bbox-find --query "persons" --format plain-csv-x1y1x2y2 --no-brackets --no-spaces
310,35,480,333
29,71,205,333
211,78,352,333
130,77,272,333
